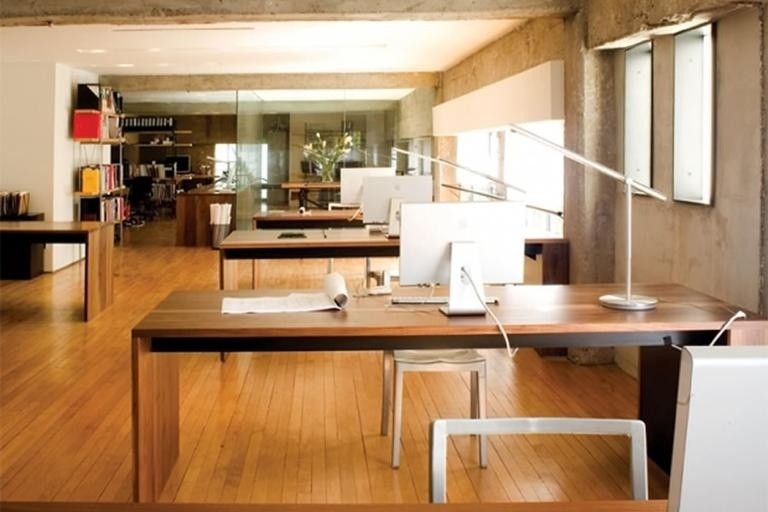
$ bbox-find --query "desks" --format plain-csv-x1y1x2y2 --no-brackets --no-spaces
176,171,221,192
250,209,366,290
218,229,570,365
281,181,340,209
0,211,116,325
130,282,766,504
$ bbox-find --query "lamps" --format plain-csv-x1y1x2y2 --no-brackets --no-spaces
510,125,669,310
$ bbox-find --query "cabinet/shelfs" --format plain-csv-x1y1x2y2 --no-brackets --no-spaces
73,83,128,246
132,130,193,148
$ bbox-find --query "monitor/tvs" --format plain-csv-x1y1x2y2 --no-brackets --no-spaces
399,200,526,316
341,167,395,216
667,346,768,512
362,175,432,238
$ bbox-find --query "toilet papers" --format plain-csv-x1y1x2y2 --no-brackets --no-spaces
297,206,313,216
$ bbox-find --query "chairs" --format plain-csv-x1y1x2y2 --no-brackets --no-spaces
363,223,400,289
420,410,656,503
373,266,490,472
327,200,372,273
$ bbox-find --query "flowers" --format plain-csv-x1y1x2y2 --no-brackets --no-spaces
291,131,354,177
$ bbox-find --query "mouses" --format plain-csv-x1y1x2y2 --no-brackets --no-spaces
367,286,392,295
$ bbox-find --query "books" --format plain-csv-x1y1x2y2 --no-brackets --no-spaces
0,189,31,218
72,81,178,226
219,271,349,315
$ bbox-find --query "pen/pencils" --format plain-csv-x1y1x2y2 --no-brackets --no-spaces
322,231,328,239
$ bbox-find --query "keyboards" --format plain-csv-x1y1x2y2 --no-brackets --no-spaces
366,225,390,233
392,296,500,304
